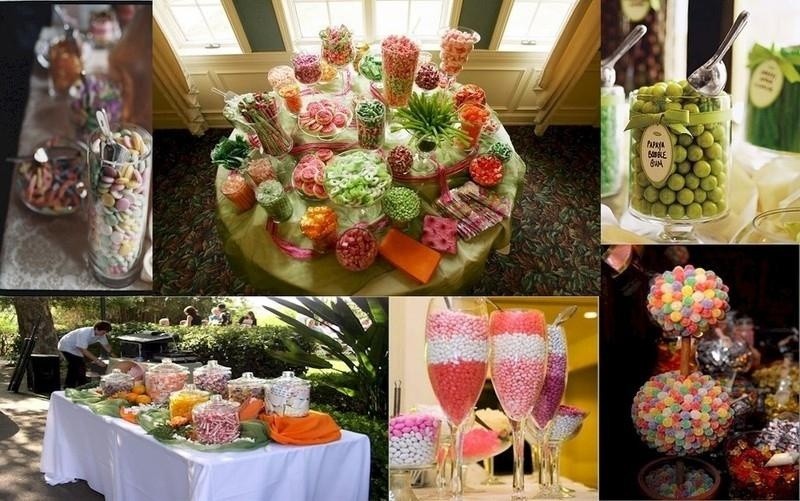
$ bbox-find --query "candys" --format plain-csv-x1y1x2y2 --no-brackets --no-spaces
16,14,150,276
601,106,619,194
628,80,727,220
632,263,800,501
388,308,587,465
220,21,511,273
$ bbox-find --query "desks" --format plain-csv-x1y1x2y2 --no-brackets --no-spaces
40,384,372,501
1,4,153,291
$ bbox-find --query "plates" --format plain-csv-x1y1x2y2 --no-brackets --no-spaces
34,38,51,73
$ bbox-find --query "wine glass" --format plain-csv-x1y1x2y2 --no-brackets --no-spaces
229,22,506,271
427,296,590,499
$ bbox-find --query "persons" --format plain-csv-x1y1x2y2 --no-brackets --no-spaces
219,304,231,326
209,307,221,324
184,306,202,327
57,322,121,389
248,311,256,326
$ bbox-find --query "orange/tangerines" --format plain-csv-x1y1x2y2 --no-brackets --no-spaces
113,384,151,404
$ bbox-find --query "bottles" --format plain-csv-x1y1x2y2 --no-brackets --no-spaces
191,395,241,446
100,369,135,397
144,358,311,422
729,209,800,245
88,2,136,48
15,138,90,218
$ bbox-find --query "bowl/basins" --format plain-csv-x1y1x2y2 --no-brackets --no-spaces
389,416,518,468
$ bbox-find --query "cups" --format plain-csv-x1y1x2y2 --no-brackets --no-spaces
50,39,83,98
723,429,799,501
389,472,418,501
67,74,124,139
85,123,153,289
601,83,628,203
628,89,733,230
219,173,255,214
